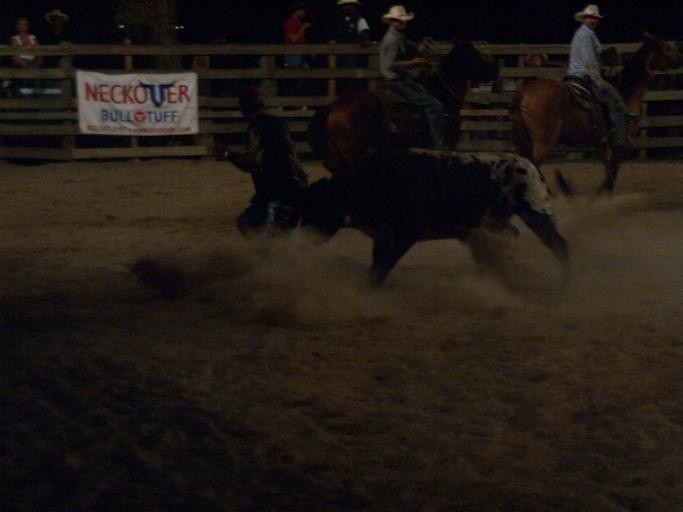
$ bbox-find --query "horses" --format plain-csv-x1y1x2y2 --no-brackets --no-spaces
309,22,501,176
510,28,683,205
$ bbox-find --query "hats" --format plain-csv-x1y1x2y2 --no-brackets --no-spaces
383,5,415,22
575,4,604,23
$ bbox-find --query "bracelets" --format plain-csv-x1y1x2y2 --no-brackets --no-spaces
224,150,229,159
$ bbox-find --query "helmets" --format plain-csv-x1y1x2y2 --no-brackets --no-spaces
238,90,264,107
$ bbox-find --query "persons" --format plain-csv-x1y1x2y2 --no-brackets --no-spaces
215,87,310,259
10,1,635,152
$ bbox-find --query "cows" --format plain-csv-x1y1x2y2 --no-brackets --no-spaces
270,150,584,293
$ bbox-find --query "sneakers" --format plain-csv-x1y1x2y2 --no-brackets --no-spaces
626,112,640,117
